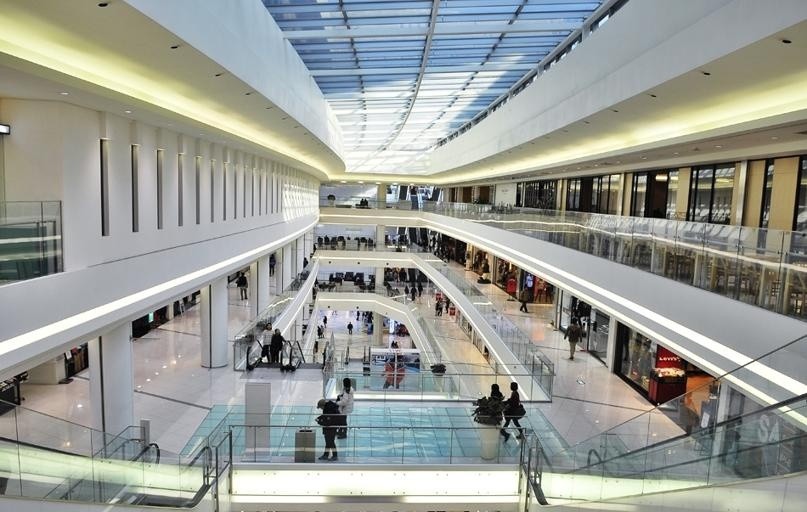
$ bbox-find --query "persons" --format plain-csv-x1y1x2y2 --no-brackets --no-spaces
525,273,552,303
564,317,582,360
491,382,526,443
356,311,365,322
519,285,530,312
404,282,424,301
315,399,339,461
236,271,248,300
317,325,325,339
262,323,286,368
323,316,327,328
270,253,276,277
336,378,353,438
434,241,450,261
380,355,406,389
437,297,450,316
347,322,353,334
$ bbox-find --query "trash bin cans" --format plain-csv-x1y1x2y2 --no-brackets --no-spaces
295,429,316,463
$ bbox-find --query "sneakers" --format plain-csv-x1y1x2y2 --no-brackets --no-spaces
336,430,346,439
505,433,510,442
516,433,525,439
318,455,337,461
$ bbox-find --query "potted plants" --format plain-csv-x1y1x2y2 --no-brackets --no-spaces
429,362,446,377
470,395,508,460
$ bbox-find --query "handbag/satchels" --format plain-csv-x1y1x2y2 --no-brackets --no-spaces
517,401,525,419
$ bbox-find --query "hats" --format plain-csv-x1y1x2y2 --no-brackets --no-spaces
317,399,325,408
